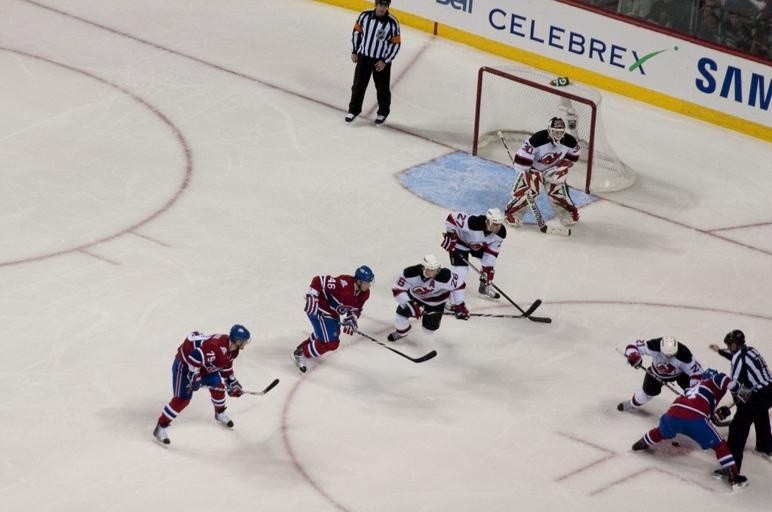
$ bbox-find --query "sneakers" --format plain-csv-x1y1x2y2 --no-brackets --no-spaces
297,341,307,348
617,399,636,412
478,281,500,299
632,441,647,450
388,330,404,341
714,466,735,476
345,110,357,122
729,472,747,484
293,348,307,373
153,422,171,444
755,444,772,457
214,409,234,427
374,110,390,124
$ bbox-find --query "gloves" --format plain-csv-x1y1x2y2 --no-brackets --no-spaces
731,382,751,406
404,300,421,320
442,234,457,252
714,405,732,422
626,350,642,370
303,292,318,317
454,305,470,321
342,314,357,336
225,380,243,398
185,371,201,392
478,269,495,284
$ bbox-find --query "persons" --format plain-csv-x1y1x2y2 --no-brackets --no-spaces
288,265,375,377
618,335,703,413
153,324,251,449
629,368,749,491
440,207,507,320
387,255,470,341
504,116,581,227
346,0,401,125
708,329,772,482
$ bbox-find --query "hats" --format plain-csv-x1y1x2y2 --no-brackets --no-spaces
374,0,391,5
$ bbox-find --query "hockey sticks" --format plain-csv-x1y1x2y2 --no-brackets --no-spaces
620,352,732,427
420,298,544,319
495,130,572,237
315,309,438,363
197,378,279,396
451,246,553,323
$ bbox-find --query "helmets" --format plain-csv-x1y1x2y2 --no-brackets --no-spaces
546,116,566,147
230,324,250,343
701,366,718,382
421,253,440,270
659,334,678,357
486,207,505,225
723,328,746,346
355,265,375,290
